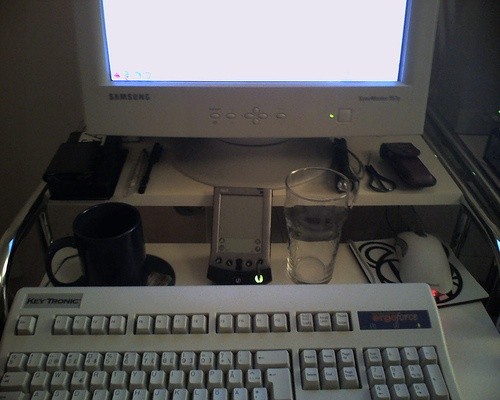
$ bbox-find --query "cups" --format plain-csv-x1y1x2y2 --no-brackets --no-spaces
284,167,352,284
43,202,148,286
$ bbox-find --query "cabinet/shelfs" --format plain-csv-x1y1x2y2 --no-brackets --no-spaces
0,128,500,396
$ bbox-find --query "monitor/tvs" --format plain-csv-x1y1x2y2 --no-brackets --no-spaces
70,0,441,187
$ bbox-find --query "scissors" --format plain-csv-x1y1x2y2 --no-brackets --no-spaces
365,153,396,193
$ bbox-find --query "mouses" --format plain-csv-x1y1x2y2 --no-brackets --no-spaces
392,228,453,297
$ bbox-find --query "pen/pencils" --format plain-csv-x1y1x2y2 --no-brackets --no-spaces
131,148,146,187
138,143,160,194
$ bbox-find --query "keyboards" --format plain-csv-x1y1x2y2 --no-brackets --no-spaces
0,281,463,400
